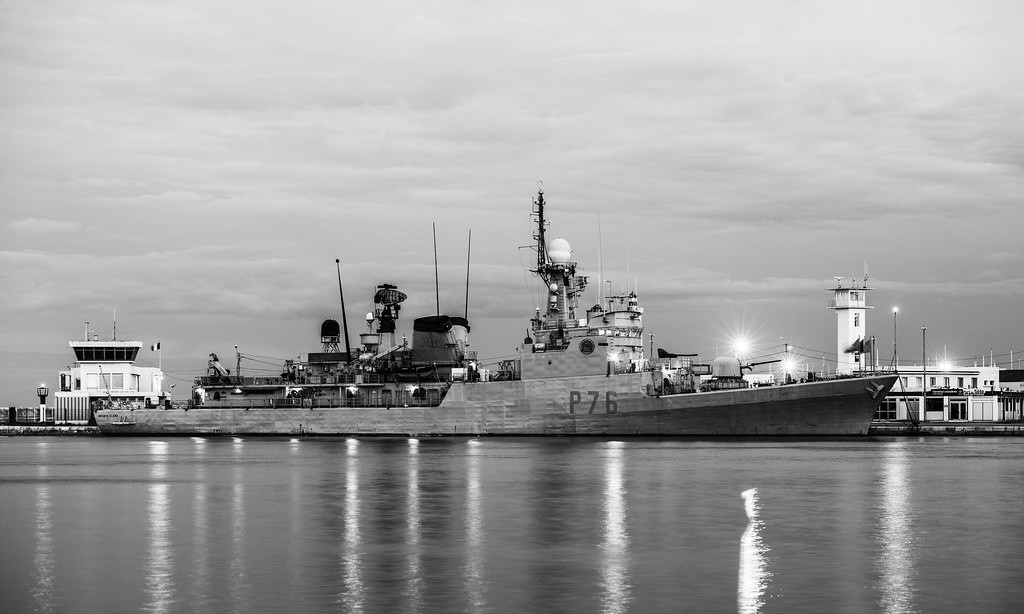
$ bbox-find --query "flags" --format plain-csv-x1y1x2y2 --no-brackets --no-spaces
844,339,871,354
151,342,160,351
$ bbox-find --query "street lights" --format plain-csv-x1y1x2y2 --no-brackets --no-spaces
921,327,927,421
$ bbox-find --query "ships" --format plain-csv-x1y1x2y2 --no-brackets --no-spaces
92,182,900,445
